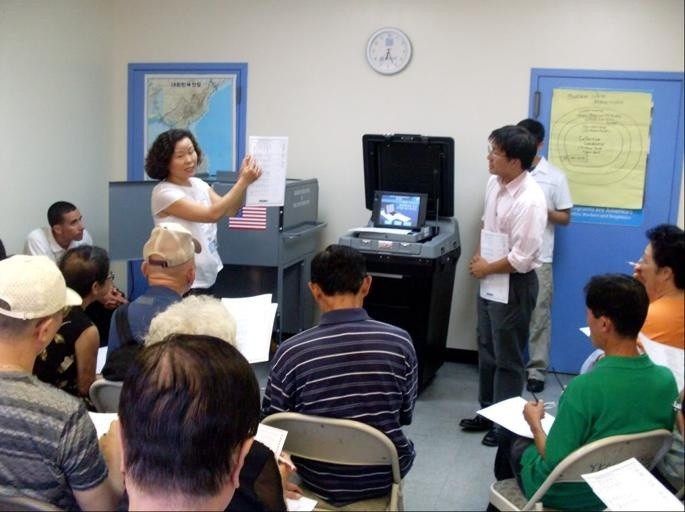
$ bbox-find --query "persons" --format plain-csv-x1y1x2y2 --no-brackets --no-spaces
140,293,304,512
23,201,129,349
103,222,202,380
458,125,549,446
672,386,685,443
142,127,263,300
517,119,574,392
485,272,680,512
30,244,116,413
0,253,125,511
116,332,262,511
260,243,422,505
578,223,685,374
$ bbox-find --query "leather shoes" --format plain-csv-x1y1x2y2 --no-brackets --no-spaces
527,379,544,392
481,431,498,446
460,416,490,431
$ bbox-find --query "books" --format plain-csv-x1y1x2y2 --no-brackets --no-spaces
245,135,288,207
479,228,510,305
219,292,279,364
475,395,557,440
578,453,685,511
228,204,267,230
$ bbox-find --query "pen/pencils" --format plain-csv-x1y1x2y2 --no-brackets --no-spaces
626,261,637,266
527,383,541,403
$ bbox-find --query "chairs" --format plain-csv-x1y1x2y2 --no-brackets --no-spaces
88,376,124,412
0,494,64,512
488,427,673,512
259,411,405,512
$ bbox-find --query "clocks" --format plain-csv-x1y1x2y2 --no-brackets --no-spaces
365,27,413,76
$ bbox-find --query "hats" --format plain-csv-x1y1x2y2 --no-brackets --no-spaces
142,221,202,267
0,253,83,321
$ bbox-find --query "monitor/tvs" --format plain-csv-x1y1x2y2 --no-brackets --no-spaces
371,190,428,229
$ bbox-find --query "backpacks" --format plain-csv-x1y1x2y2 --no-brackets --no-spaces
100,303,146,380
